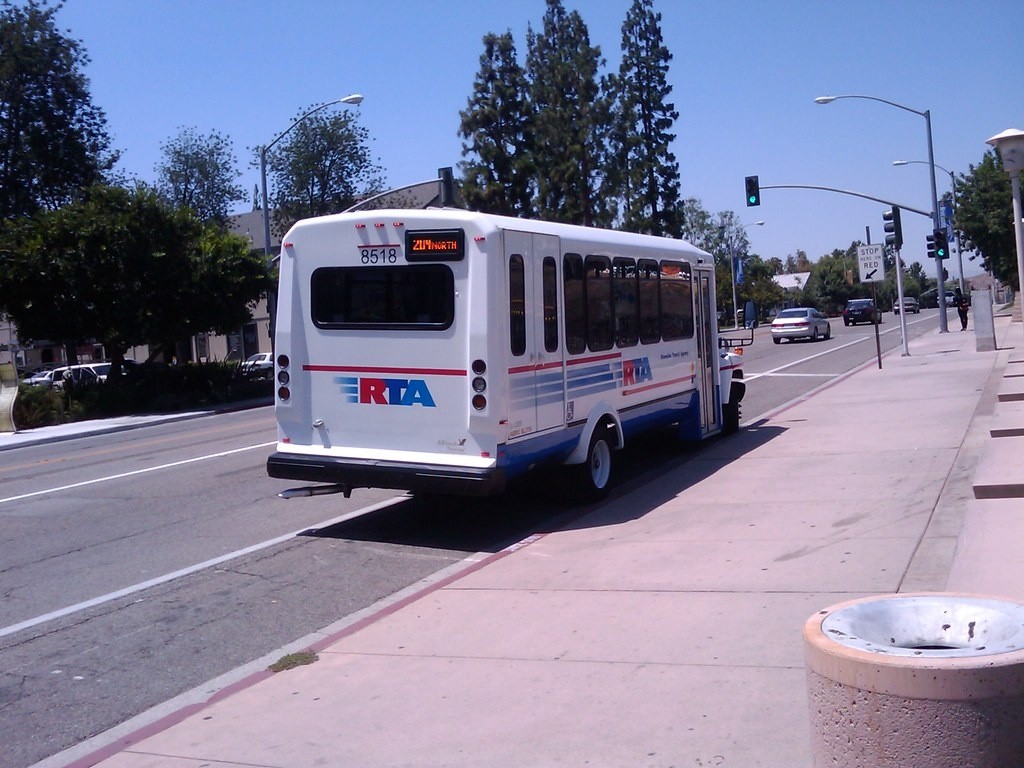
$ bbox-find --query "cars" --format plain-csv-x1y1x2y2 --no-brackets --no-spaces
240,352,274,380
937,291,957,306
893,297,919,314
21,357,134,393
843,298,882,326
770,307,830,344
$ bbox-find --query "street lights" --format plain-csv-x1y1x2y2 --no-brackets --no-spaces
727,219,766,332
892,160,965,299
813,94,948,334
259,94,368,264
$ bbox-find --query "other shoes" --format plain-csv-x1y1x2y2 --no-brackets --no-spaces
961,327,966,331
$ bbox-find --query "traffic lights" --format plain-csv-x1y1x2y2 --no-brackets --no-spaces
880,205,903,246
932,227,949,260
926,234,935,258
745,174,761,207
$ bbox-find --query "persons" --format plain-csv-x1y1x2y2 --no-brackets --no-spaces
61,367,73,382
953,288,969,331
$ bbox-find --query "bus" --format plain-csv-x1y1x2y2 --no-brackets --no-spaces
263,207,760,505
